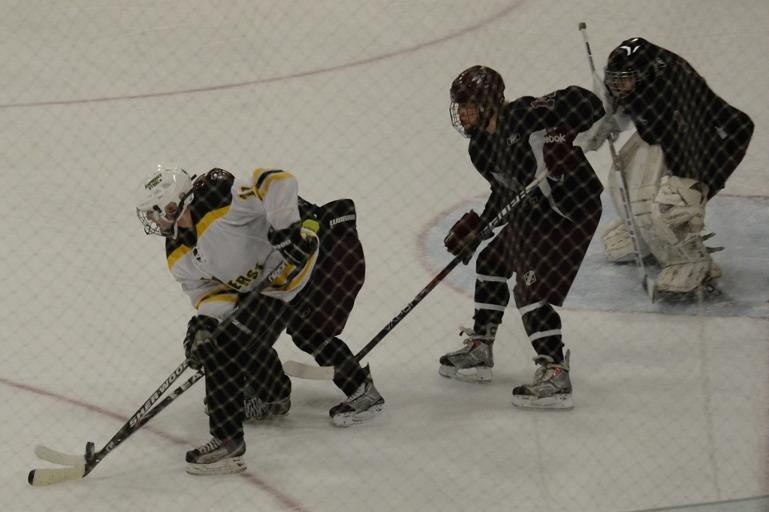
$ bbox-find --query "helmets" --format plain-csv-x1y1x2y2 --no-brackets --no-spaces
131,164,196,243
601,35,655,100
447,65,505,140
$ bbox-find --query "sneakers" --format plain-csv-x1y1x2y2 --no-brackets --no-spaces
185,434,247,464
512,349,572,397
439,325,495,368
329,361,384,418
202,398,292,420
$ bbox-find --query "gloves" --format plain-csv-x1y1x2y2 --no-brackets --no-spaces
542,129,577,173
180,316,220,369
266,223,317,267
443,208,494,263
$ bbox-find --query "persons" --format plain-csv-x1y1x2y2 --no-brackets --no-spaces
438,65,604,409
136,164,384,476
605,37,754,296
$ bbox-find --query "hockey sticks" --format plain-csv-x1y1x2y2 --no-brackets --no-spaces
282,169,551,379
35,364,210,465
28,233,312,487
28,259,288,486
579,23,656,304
